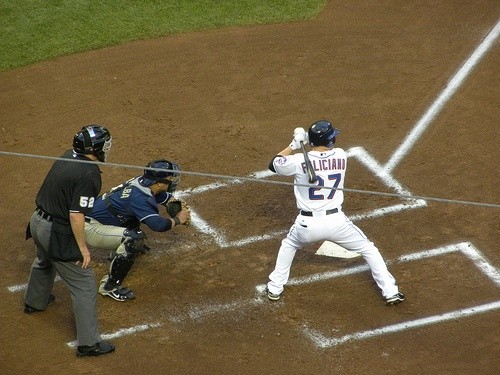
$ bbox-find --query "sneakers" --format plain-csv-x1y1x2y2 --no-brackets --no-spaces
264,286,281,300
385,291,405,305
99,276,136,302
77,341,116,357
24,295,56,314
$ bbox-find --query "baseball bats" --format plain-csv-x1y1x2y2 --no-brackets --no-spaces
301,142,315,183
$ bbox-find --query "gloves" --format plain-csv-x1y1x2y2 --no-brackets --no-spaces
289,127,310,151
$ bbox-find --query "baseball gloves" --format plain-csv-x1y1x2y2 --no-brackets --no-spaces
165,197,191,218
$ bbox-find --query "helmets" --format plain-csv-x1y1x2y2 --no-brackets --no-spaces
73,125,111,163
310,121,339,147
139,159,179,187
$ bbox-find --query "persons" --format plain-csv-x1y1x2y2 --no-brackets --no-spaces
24,125,115,357
266,121,406,307
85,159,191,302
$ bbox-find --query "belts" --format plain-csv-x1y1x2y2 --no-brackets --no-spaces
301,208,340,216
35,206,54,223
